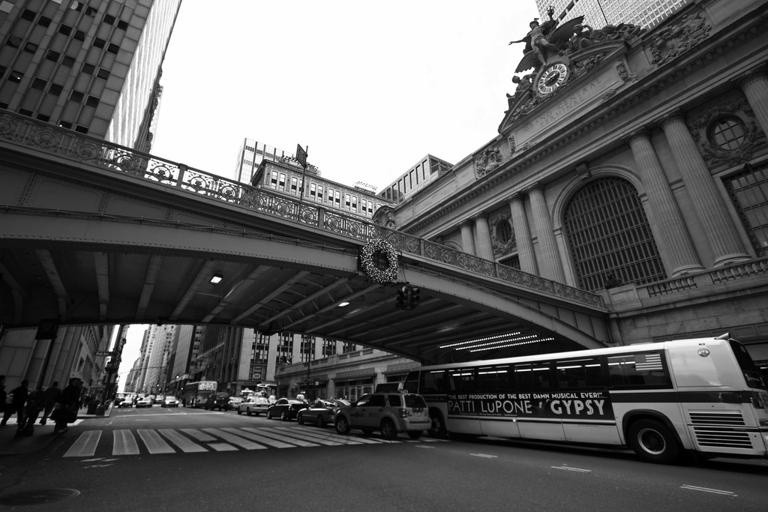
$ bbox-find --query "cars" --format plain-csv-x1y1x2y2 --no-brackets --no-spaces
115,392,179,408
204,389,432,442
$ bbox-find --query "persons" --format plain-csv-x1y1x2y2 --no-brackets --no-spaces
504,9,635,115
0,374,91,440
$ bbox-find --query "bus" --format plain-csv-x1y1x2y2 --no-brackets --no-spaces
181,381,217,408
403,333,768,462
256,384,277,396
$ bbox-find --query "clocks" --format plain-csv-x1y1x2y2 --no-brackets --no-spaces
536,62,567,94
